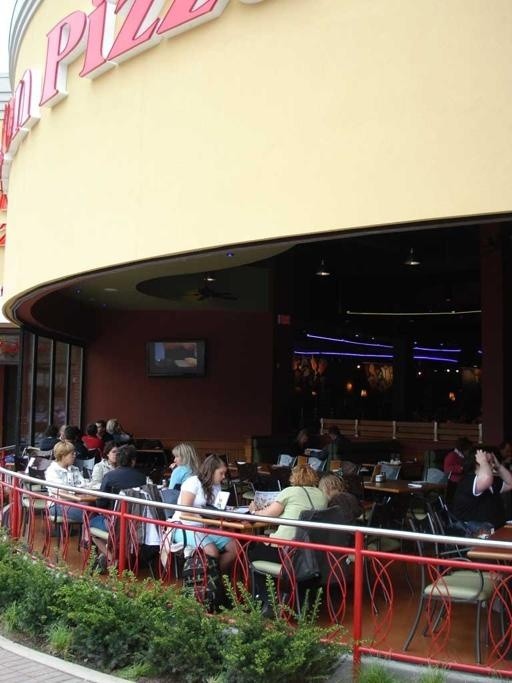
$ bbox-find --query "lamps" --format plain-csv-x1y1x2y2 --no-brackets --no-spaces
315,255,330,277
400,248,423,267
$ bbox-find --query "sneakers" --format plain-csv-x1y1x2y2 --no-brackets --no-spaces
108,558,122,576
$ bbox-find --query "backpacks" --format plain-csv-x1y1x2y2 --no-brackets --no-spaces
183,546,231,617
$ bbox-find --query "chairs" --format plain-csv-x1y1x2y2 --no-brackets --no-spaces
3,436,388,665
397,491,512,665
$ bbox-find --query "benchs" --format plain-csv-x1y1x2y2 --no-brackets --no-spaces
247,437,501,484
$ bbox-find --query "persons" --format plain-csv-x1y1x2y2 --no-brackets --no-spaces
1,418,153,574
287,426,353,462
161,441,205,491
167,455,240,612
247,463,369,620
443,437,511,613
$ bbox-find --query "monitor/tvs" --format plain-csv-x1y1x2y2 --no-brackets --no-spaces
145,337,207,378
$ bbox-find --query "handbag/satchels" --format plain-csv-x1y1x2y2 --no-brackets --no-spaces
448,520,494,541
281,531,320,590
